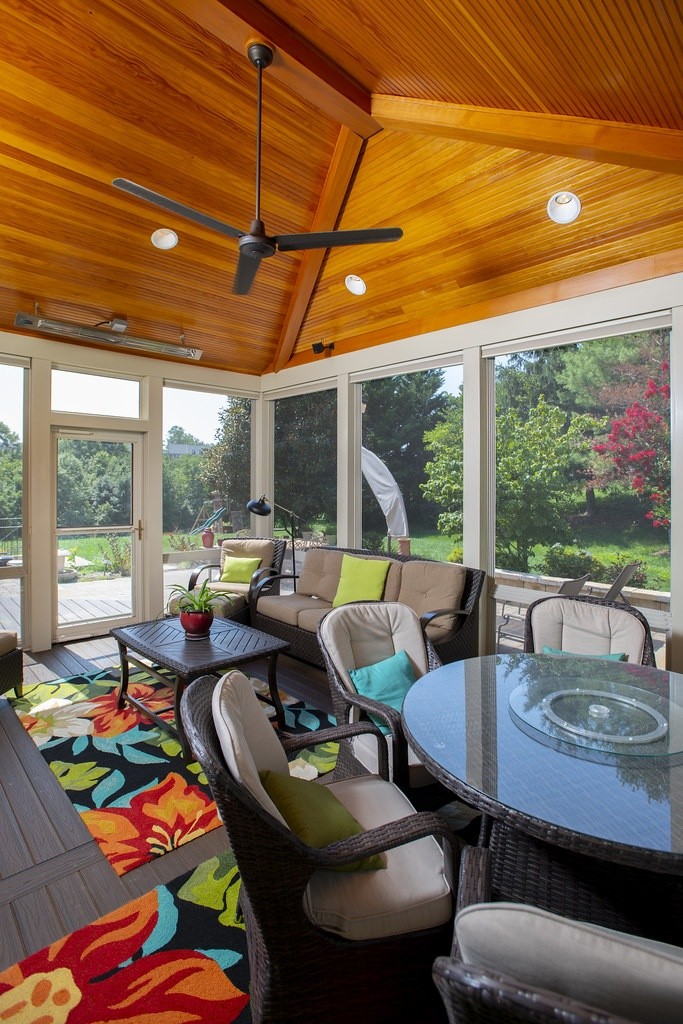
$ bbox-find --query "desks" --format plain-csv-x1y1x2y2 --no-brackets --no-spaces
401,653,683,950
110,614,291,761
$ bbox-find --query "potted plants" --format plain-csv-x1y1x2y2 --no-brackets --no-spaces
153,578,249,640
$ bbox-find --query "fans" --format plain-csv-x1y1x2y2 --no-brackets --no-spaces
112,46,403,296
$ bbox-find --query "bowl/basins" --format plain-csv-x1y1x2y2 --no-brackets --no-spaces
57,568,77,581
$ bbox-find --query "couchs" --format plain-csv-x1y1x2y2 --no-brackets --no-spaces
249,546,485,669
165,538,287,620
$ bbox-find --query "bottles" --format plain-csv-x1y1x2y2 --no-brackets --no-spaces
202,527,214,548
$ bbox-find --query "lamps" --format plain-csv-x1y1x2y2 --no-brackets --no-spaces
14,303,203,360
246,494,307,593
312,342,334,354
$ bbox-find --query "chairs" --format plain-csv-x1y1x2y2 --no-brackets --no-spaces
495,573,592,652
523,595,656,669
179,673,461,1024
431,817,683,1024
317,600,444,792
603,561,644,606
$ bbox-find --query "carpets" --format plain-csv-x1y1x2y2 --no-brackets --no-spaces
0,846,254,1024
4,657,340,876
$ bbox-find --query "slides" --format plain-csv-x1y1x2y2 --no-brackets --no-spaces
190,507,227,535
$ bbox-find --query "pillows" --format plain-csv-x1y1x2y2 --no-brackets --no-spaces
333,554,390,608
257,769,385,872
348,649,417,735
220,556,262,584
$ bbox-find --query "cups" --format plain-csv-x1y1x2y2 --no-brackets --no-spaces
218,539,224,545
